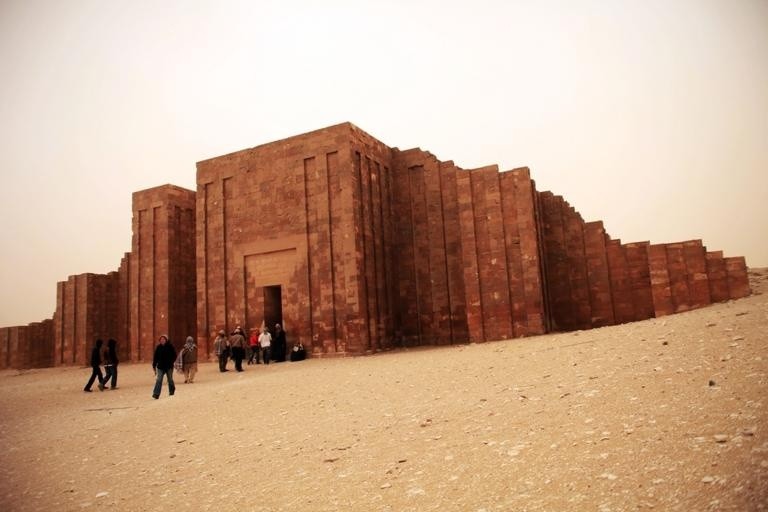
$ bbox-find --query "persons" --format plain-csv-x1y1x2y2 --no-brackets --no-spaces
98,337,119,392
291,343,306,361
214,324,286,372
84,338,109,392
181,336,198,383
152,335,177,399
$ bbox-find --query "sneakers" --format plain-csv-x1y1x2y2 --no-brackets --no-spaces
152,394,159,399
170,390,174,395
84,387,92,392
98,383,118,391
